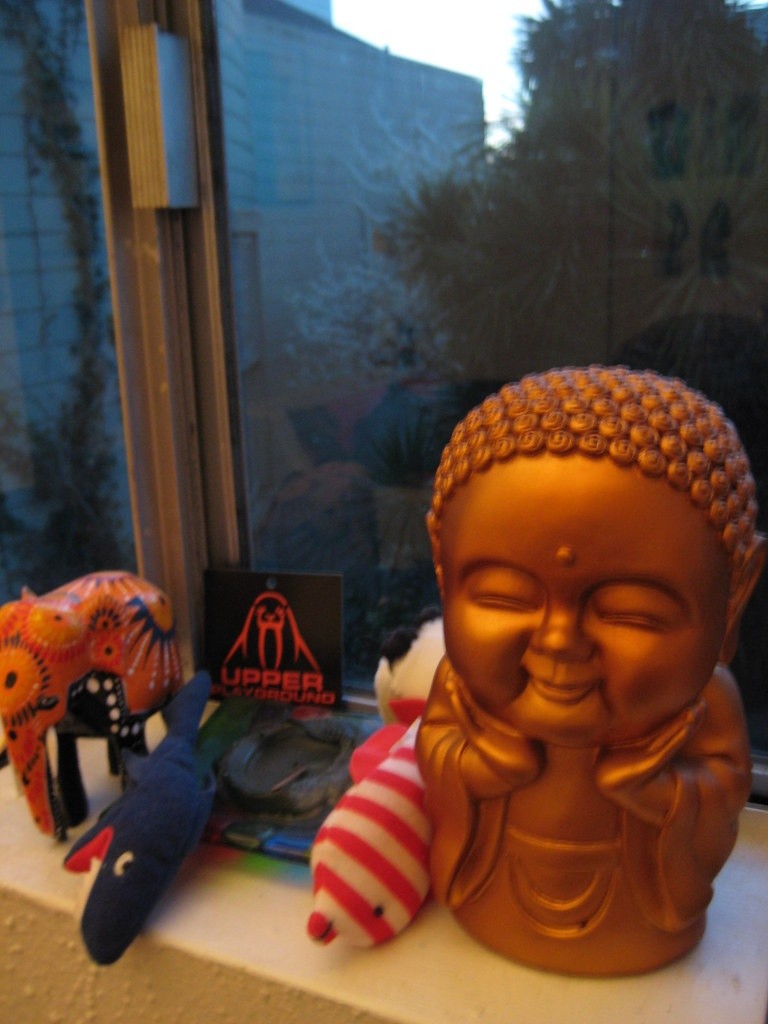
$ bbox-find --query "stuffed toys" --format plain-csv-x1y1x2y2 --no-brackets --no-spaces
308,606,452,950
64,669,215,965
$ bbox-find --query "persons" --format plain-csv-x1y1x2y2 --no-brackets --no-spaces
414,366,768,978
